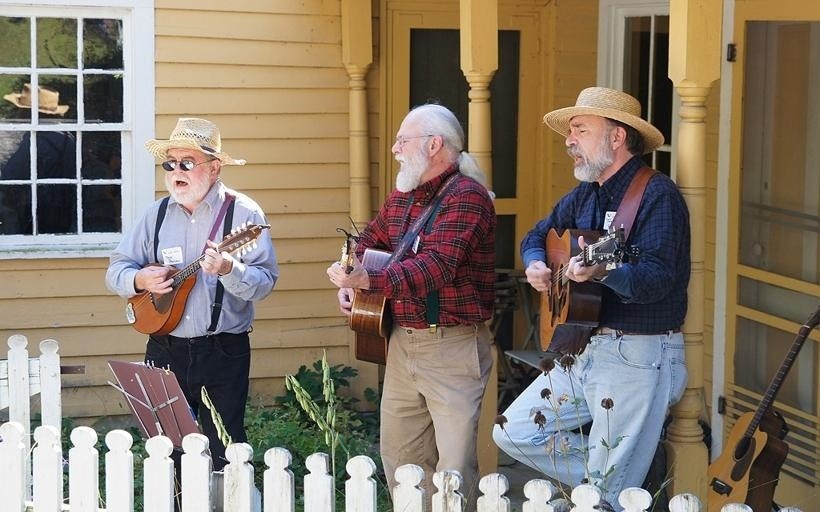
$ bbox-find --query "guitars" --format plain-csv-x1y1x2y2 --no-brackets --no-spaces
708,300,820,512
540,225,640,356
336,228,395,367
125,220,272,336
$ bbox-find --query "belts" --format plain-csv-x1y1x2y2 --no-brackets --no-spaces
594,327,680,336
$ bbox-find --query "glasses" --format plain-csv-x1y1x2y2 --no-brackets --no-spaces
393,135,433,145
162,158,217,171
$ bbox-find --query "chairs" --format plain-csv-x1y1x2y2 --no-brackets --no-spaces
498,270,588,418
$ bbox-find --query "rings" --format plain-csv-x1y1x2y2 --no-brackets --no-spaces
332,277,335,282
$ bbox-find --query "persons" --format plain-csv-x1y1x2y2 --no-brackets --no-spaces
105,117,279,511
492,87,689,512
0,82,103,233
326,105,496,512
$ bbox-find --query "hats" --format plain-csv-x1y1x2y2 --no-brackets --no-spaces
542,86,665,155
3,84,70,115
145,118,247,166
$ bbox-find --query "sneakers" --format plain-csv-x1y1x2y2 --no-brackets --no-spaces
642,442,669,512
